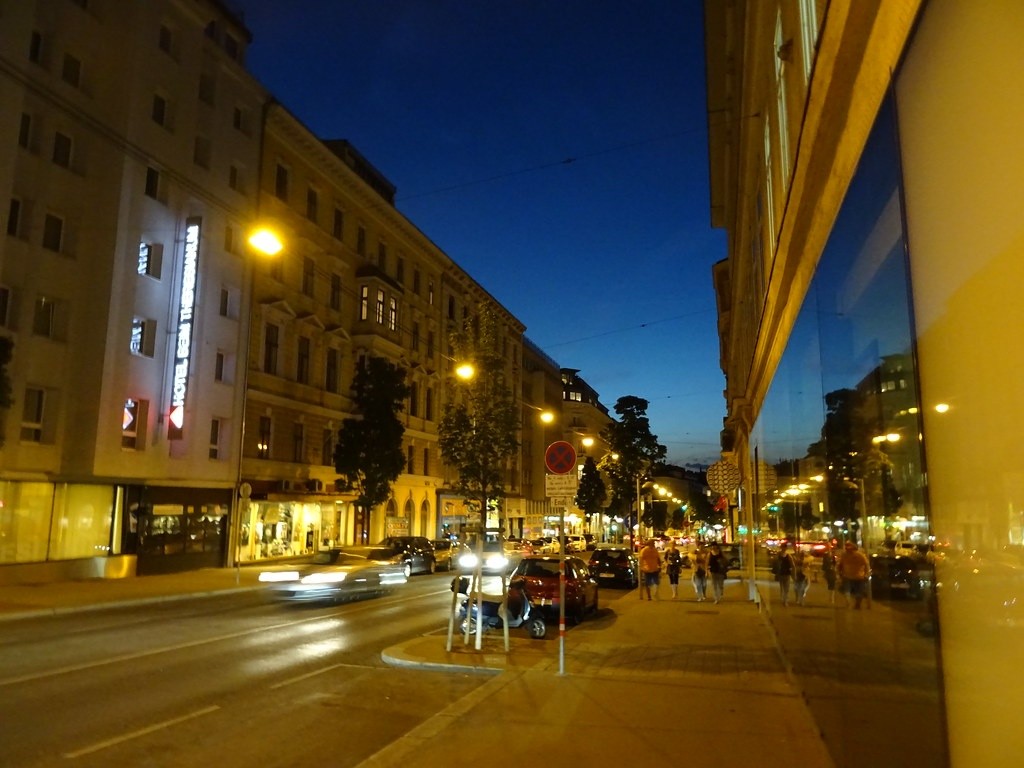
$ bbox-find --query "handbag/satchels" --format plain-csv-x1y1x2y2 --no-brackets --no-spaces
717,555,730,569
771,561,781,574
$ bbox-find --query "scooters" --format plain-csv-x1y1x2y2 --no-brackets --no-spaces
450,563,547,640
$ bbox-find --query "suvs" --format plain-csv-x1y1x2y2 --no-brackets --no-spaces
910,544,933,562
894,541,918,557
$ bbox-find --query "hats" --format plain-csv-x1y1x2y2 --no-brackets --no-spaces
708,541,718,547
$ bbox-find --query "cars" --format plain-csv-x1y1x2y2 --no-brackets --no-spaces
662,536,689,546
587,547,638,589
367,537,436,578
429,539,472,572
557,536,575,553
926,545,946,566
634,538,665,552
868,553,924,599
758,547,779,567
568,534,597,552
269,545,407,606
538,537,560,554
480,538,533,573
529,540,550,555
716,543,741,570
506,554,598,625
800,542,828,556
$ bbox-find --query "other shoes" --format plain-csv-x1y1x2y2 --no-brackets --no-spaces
648,595,652,601
796,597,800,604
703,596,707,600
715,599,719,604
655,591,660,597
783,600,788,606
800,599,805,607
672,592,676,598
698,597,702,601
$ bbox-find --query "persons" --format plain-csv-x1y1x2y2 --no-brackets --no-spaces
772,540,872,610
664,540,682,600
639,540,661,601
690,542,709,603
705,541,728,604
